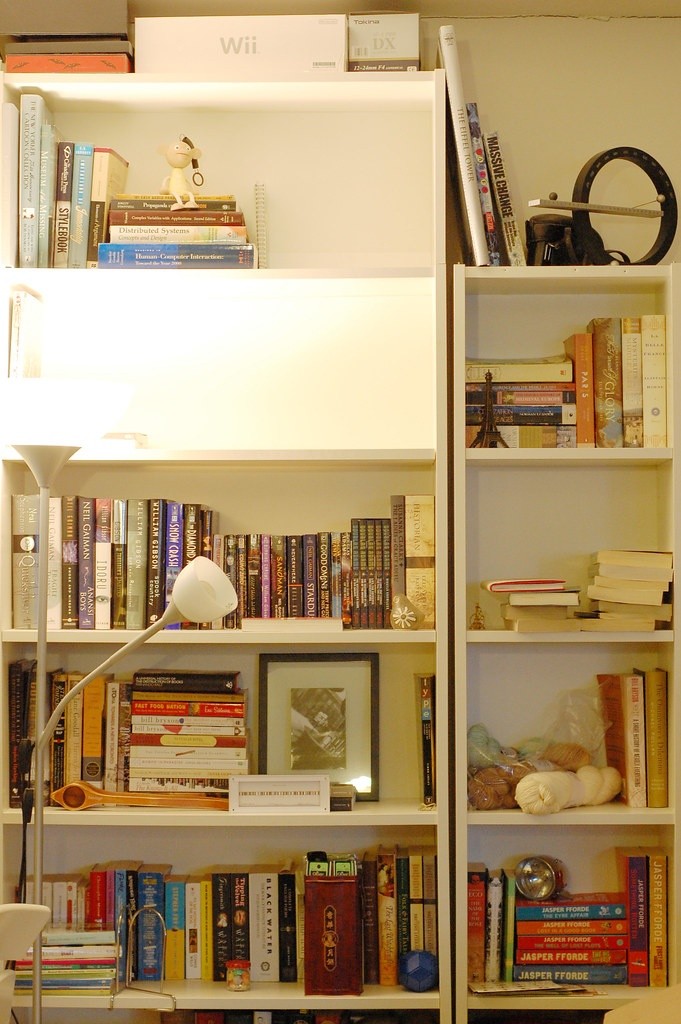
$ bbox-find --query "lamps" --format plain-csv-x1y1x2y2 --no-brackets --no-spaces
11,445,239,1024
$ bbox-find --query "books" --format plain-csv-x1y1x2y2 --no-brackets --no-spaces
466,315,668,448
467,846,668,987
416,673,435,810
8,657,248,808
481,549,676,633
595,667,668,809
6,845,438,994
2,94,274,268
438,26,529,267
11,494,434,630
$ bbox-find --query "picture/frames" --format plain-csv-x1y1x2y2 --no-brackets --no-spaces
258,652,380,802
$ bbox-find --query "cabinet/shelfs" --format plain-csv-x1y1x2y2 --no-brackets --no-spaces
0,68,681,1024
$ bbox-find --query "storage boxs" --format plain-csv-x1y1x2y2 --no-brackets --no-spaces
347,10,421,72
0,0,134,74
304,873,364,997
134,14,349,74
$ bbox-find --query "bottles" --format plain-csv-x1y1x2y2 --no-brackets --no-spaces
225,960,251,991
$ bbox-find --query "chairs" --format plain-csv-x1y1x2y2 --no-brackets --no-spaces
0,903,51,1024
